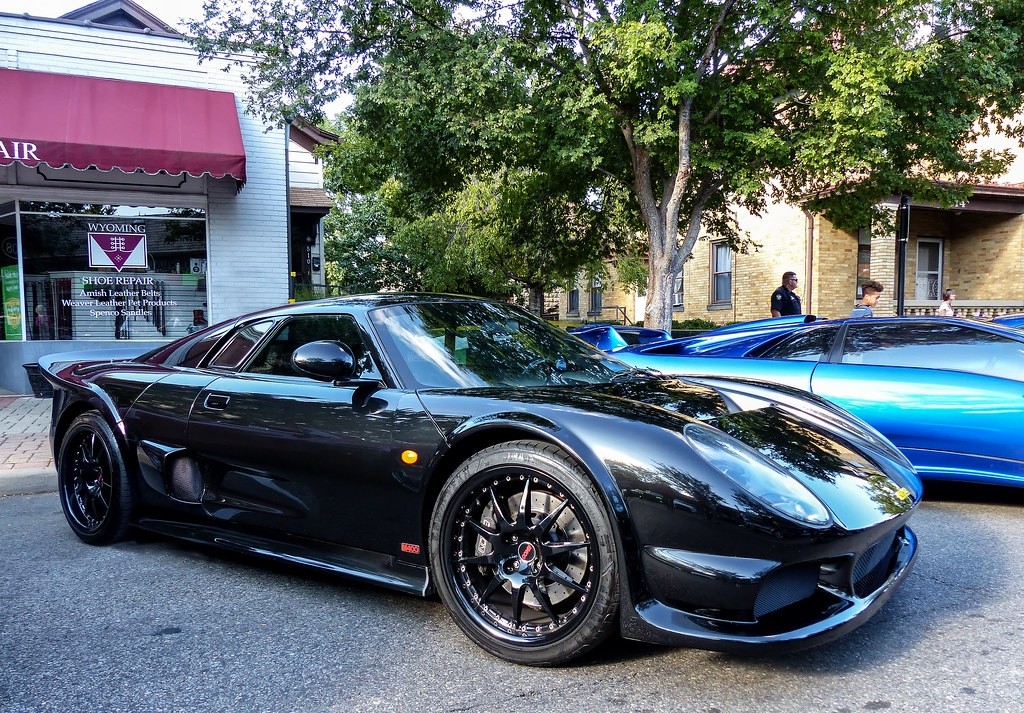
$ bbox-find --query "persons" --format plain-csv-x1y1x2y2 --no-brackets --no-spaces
939,289,957,316
849,281,883,320
36,305,54,341
771,272,804,318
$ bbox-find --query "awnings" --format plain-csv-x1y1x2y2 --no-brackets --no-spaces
0,68,248,197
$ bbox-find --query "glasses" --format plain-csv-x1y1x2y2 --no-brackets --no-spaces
790,279,797,282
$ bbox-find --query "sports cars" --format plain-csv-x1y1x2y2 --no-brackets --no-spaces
36,290,929,670
564,310,1024,490
